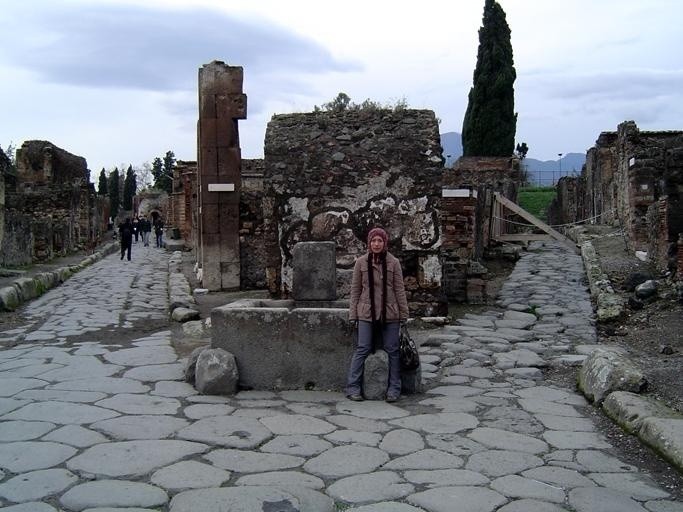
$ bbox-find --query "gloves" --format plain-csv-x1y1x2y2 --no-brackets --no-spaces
400,320,408,327
349,319,357,326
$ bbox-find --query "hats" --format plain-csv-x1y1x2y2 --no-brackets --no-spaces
367,228,388,254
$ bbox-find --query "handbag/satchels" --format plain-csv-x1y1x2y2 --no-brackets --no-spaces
398,325,420,371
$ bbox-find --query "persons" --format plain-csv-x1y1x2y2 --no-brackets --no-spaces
108,212,163,261
347,227,409,402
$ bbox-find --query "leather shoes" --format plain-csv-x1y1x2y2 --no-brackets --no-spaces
347,393,362,401
385,395,398,402
